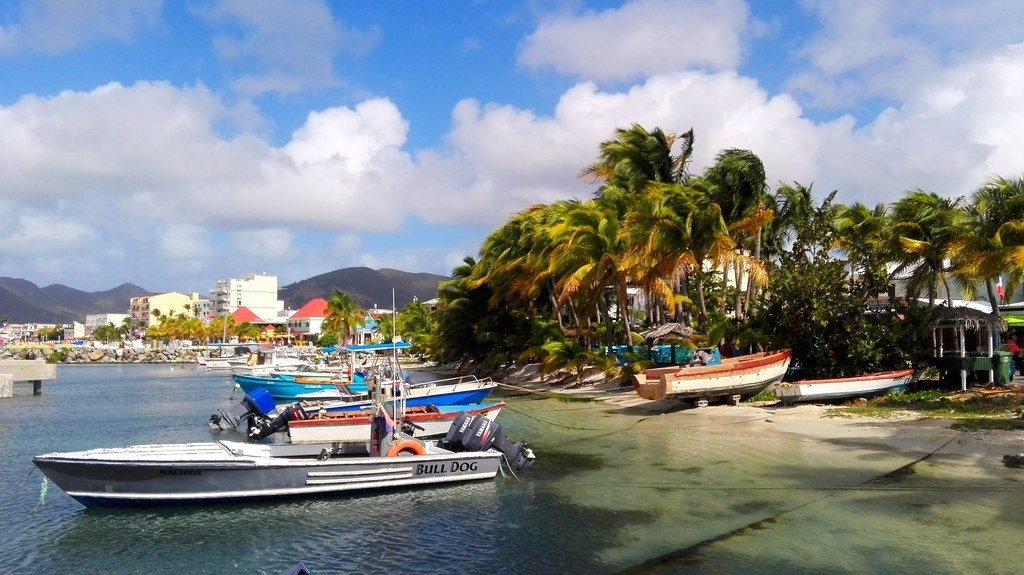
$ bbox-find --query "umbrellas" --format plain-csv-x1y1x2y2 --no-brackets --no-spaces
640,318,699,363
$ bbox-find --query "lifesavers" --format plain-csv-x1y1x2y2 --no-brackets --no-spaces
386,438,426,456
347,364,351,381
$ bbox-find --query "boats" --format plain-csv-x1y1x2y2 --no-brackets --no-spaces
774,369,916,404
630,347,792,401
196,271,508,446
31,288,537,508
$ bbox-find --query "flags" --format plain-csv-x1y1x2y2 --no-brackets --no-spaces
996,274,1004,299
